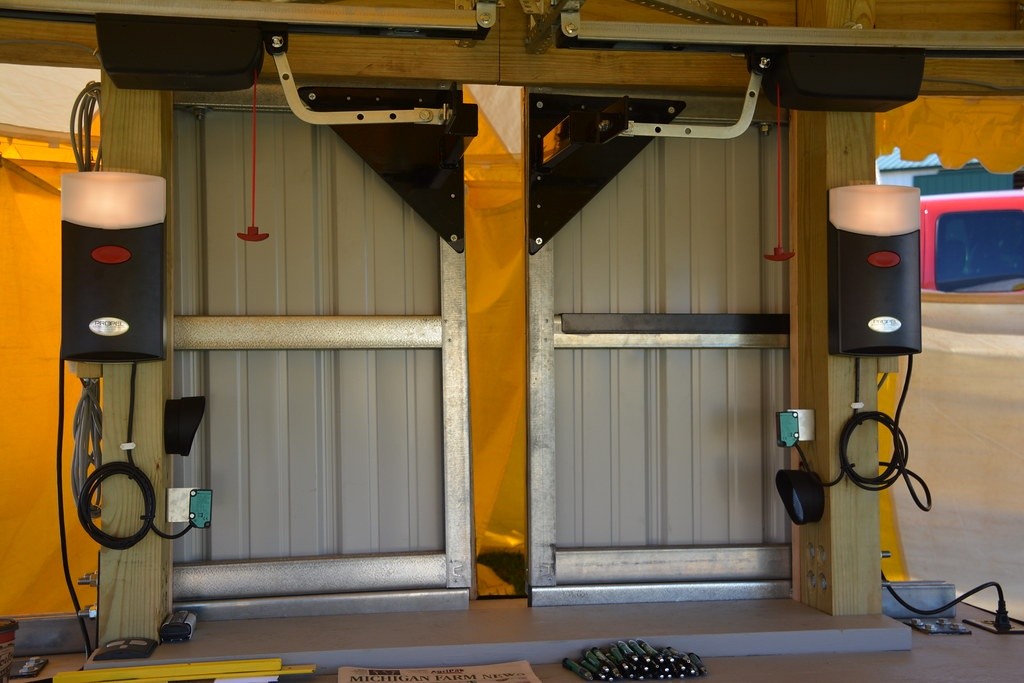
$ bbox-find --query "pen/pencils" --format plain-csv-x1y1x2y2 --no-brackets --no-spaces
561,638,708,682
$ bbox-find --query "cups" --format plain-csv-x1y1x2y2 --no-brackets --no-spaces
0,619,20,683
